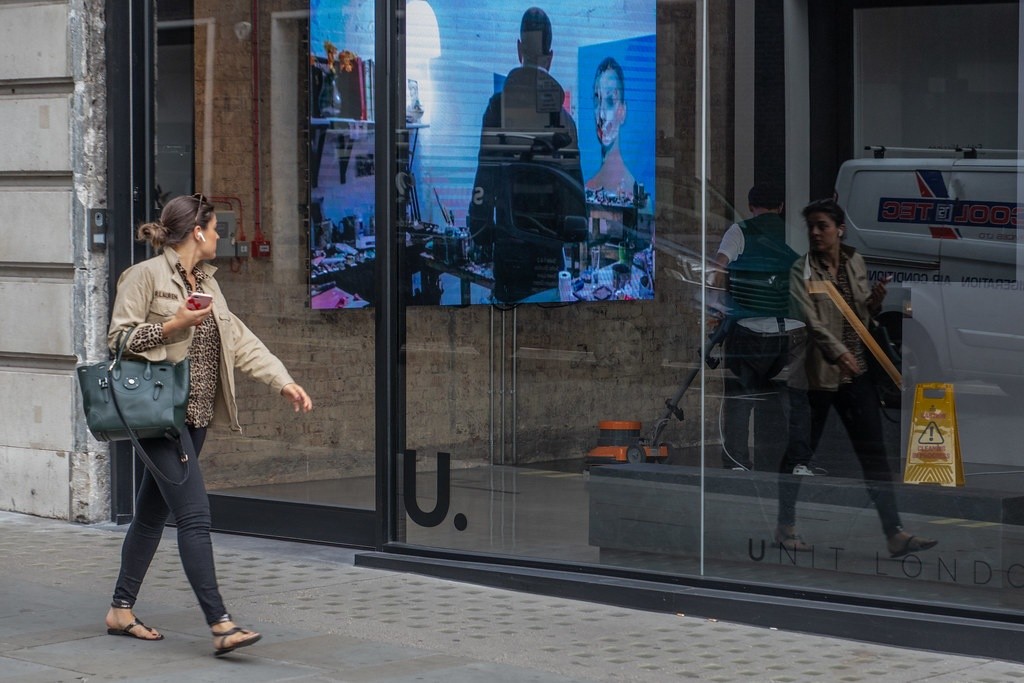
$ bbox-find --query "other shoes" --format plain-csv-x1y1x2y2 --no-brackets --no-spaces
729,457,752,471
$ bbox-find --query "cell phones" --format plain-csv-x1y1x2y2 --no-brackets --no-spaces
185,292,211,310
866,276,893,301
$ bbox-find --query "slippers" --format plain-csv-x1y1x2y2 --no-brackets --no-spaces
208,614,261,656
771,521,814,551
107,599,164,640
886,524,938,557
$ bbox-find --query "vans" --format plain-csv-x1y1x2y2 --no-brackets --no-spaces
833,145,1024,406
309,117,800,373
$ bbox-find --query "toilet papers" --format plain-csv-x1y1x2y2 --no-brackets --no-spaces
558,271,572,301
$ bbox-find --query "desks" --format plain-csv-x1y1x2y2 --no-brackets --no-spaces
525,245,653,301
308,219,474,307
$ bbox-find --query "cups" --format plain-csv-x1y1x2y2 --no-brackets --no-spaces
638,276,654,299
611,264,632,290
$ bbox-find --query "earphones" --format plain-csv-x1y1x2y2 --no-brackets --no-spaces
838,228,843,237
198,231,206,242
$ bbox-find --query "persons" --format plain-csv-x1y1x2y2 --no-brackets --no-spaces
469,7,588,301
105,192,312,655
772,198,938,555
715,184,802,468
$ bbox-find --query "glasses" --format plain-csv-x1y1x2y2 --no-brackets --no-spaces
191,193,207,224
805,198,844,214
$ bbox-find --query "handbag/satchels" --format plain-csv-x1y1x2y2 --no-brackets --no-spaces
77,327,190,486
862,318,900,422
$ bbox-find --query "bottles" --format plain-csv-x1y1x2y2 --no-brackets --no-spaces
619,177,629,203
445,220,468,259
633,180,644,207
369,215,376,237
354,213,364,238
619,241,627,264
